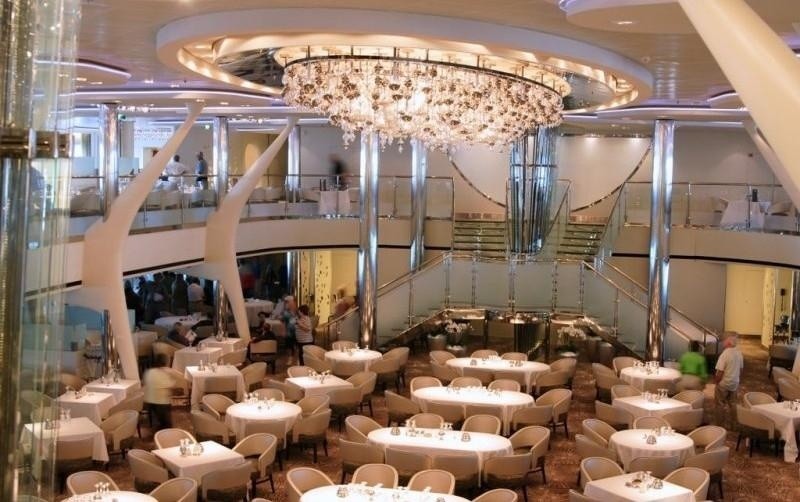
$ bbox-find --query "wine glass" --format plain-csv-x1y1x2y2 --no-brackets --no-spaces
640,388,669,403
179,438,203,457
61,408,73,432
199,359,219,376
634,361,660,375
354,342,370,354
794,398,800,411
242,390,273,416
392,419,470,443
308,368,331,383
91,481,115,501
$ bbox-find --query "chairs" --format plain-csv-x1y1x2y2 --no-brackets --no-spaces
0,290,800,502
763,199,795,236
710,195,730,224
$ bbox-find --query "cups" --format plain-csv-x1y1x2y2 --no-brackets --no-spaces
446,384,503,398
334,480,445,502
636,469,663,490
644,422,677,445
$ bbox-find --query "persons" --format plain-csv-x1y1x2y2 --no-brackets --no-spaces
675,339,709,385
123,258,323,374
714,334,746,431
165,155,189,187
327,151,349,190
193,150,210,187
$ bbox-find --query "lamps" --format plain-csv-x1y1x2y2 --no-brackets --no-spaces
272,45,571,160
